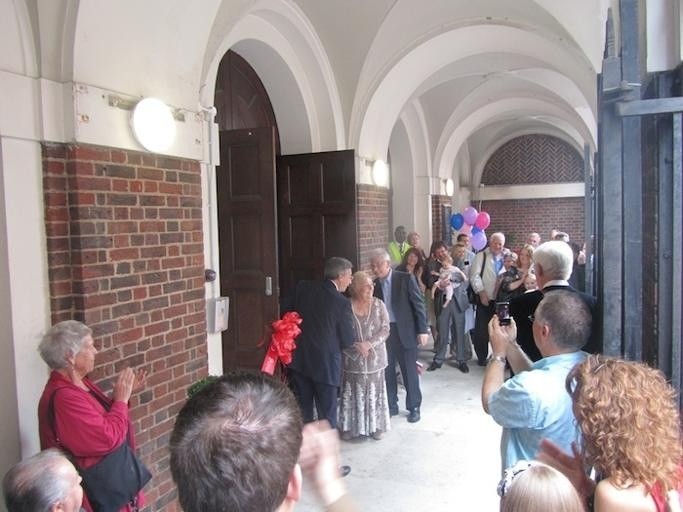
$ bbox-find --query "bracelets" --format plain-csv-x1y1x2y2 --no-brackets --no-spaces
487,351,506,366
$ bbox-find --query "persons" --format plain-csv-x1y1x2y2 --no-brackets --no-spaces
37,321,149,512
281,255,356,428
367,248,430,423
503,240,600,376
386,226,586,372
499,458,585,510
479,288,595,478
532,352,680,511
335,270,391,442
169,368,352,511
3,446,84,512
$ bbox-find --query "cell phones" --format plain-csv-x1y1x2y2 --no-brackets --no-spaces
495,301,511,325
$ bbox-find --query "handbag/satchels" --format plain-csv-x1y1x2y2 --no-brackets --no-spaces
466,285,479,305
80,442,152,512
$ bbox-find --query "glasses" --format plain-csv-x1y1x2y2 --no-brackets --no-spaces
528,315,539,323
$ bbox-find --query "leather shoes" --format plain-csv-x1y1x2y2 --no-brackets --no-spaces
389,406,399,416
408,406,419,422
338,466,351,477
342,431,351,441
373,430,384,440
426,353,487,372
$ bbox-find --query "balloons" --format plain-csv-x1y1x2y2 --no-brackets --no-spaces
451,206,489,254
